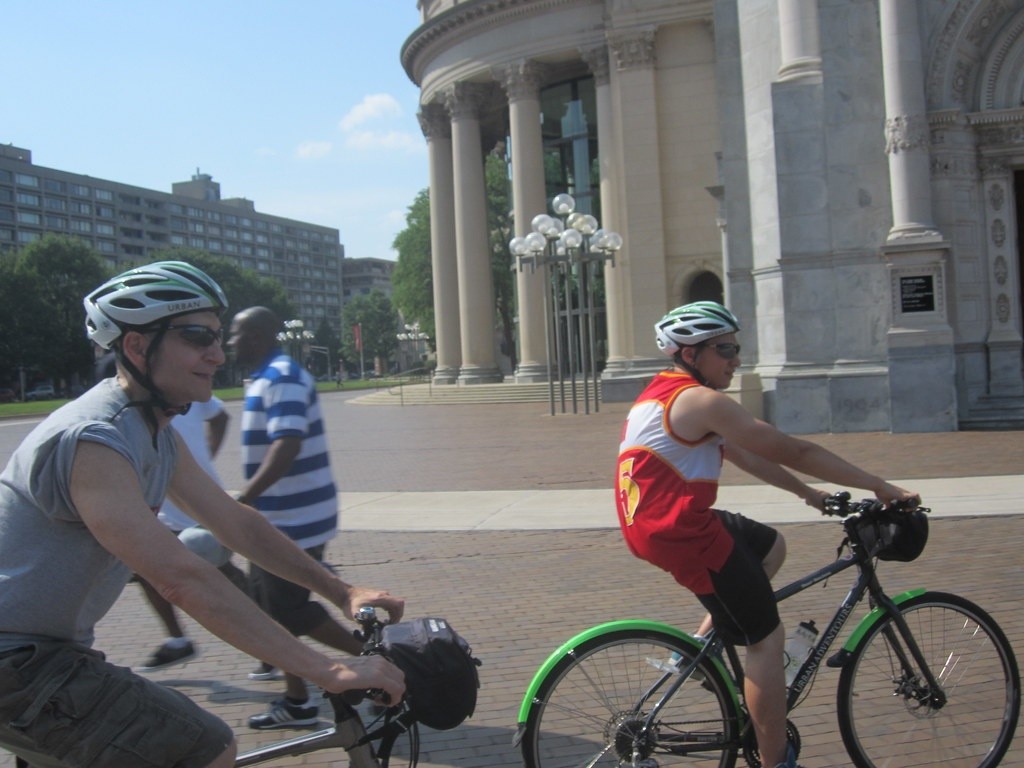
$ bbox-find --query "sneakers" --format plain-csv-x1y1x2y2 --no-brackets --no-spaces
248,660,279,681
668,634,743,695
248,696,318,730
133,636,196,672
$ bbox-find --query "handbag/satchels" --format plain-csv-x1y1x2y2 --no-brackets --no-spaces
872,507,929,563
380,615,479,730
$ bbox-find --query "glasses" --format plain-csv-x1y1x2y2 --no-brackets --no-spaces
700,342,740,358
133,326,225,347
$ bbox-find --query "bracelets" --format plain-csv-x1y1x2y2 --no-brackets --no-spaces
236,493,252,509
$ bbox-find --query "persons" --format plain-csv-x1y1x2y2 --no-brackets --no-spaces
133,384,282,680
225,304,341,732
614,301,924,768
0,258,406,767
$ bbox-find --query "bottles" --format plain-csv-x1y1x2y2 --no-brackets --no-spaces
783,620,819,702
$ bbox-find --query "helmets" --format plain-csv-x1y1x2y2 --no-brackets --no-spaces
652,301,740,357
82,260,228,350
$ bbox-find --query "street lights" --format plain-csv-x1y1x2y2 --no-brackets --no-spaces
508,193,625,417
276,319,315,368
396,322,430,385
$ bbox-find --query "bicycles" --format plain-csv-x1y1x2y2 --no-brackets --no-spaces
15,600,482,768
507,490,1021,768
